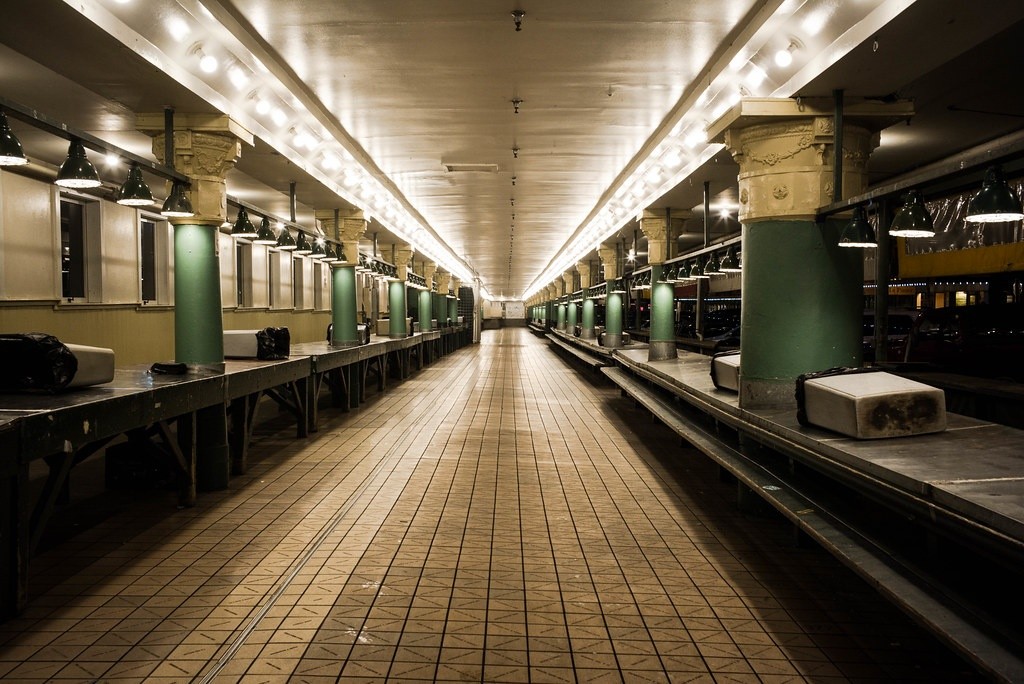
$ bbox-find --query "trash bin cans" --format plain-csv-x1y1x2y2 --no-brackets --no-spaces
431,318,452,327
709,349,741,393
0,332,115,396
458,316,464,322
796,364,949,440
598,331,631,346
327,323,367,346
575,326,600,337
223,327,291,359
413,322,419,331
376,316,415,336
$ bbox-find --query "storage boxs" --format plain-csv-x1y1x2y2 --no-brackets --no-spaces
795,364,948,440
598,330,631,346
531,317,546,324
574,326,601,338
376,317,415,336
222,327,291,360
710,349,741,391
326,323,372,345
0,332,116,393
413,316,466,332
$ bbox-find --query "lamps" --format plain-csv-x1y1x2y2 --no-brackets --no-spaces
962,167,1024,222
320,240,338,262
688,256,711,278
54,140,104,189
703,251,726,275
160,180,195,218
655,264,675,283
275,226,297,249
677,259,697,280
1,109,30,167
116,165,157,207
718,245,742,272
253,218,278,245
889,188,936,238
554,270,652,307
355,253,461,300
306,237,327,258
666,262,684,282
331,243,349,264
838,206,879,248
230,207,257,237
292,231,313,255
